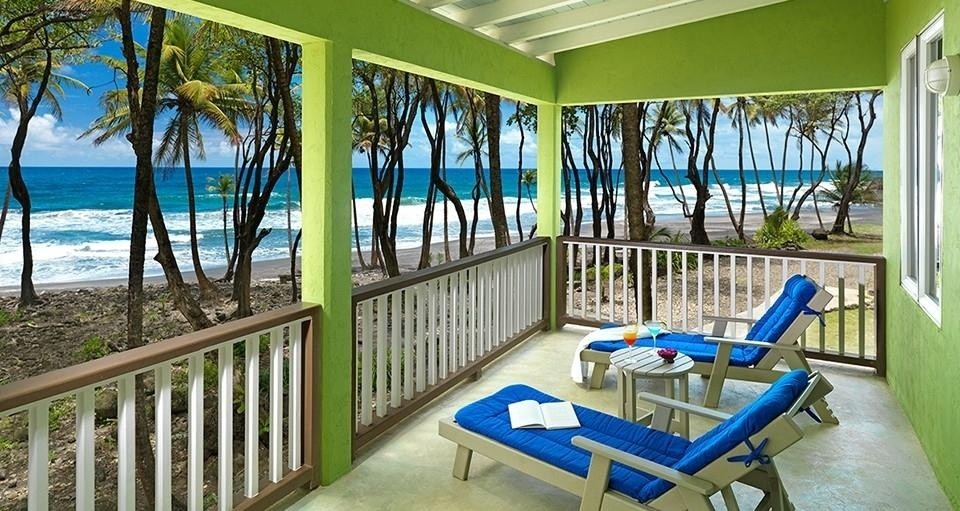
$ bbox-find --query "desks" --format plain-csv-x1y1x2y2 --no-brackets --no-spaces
609,345,694,441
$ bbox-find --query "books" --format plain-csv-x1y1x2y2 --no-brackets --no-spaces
508,399,581,430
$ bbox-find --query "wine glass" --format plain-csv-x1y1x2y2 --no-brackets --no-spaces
644,319,664,356
622,325,639,364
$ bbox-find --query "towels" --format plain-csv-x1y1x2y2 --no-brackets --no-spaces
570,317,673,384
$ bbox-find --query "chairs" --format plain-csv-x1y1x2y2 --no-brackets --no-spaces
579,272,841,425
437,368,844,511
